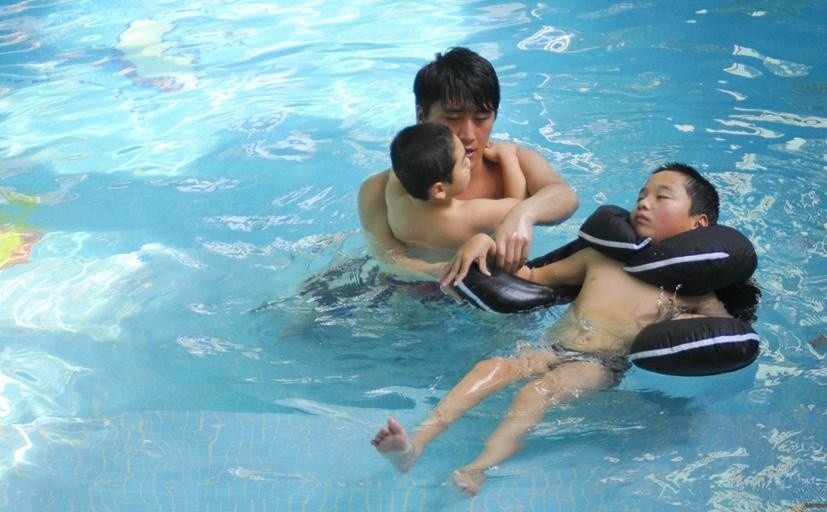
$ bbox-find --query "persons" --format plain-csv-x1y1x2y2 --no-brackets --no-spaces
383,120,528,257
370,163,735,494
281,48,579,329
